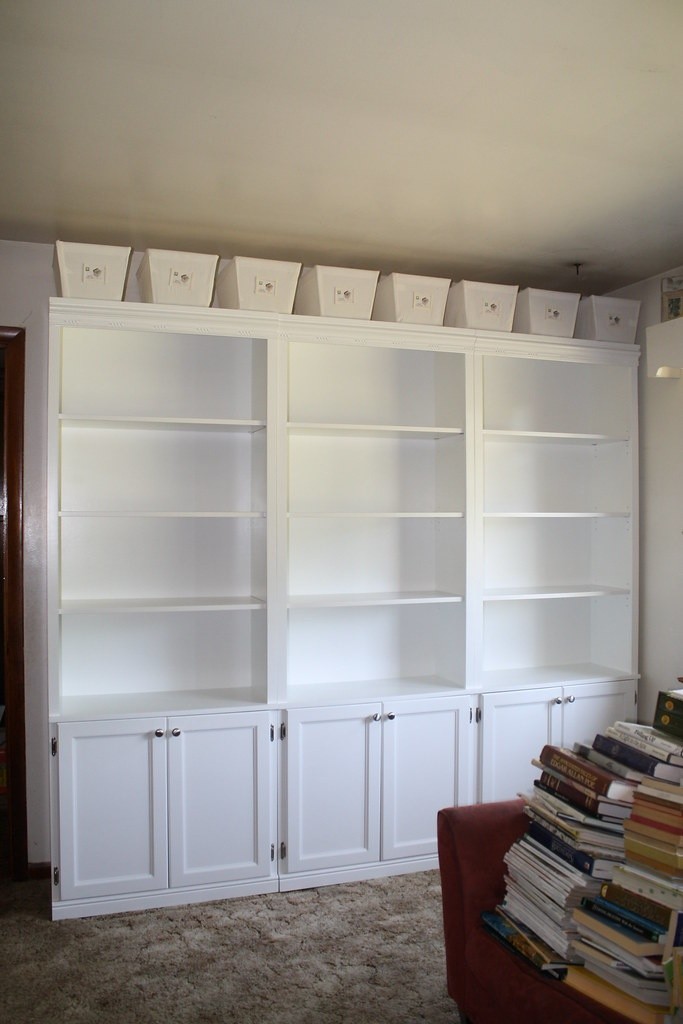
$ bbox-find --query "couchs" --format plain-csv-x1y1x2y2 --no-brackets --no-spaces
437,798,640,1024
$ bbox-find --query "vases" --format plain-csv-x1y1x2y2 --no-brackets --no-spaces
443,279,518,332
573,295,642,345
293,265,380,320
512,287,581,340
135,248,219,307
51,240,132,301
216,256,302,314
370,272,452,327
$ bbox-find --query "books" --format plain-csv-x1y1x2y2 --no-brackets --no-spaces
483,690,683,1023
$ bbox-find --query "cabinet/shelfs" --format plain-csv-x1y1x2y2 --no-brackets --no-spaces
48,296,279,923
281,314,480,891
477,330,640,803
